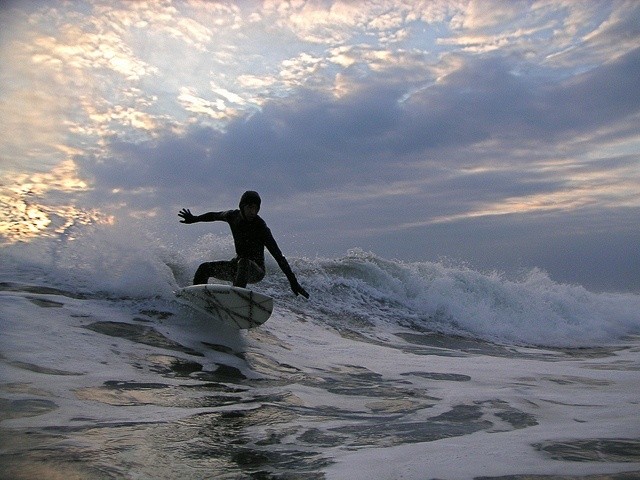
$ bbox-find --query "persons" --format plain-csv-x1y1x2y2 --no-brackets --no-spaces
177,190,311,298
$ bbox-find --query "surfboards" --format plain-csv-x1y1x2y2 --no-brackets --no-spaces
177,283,273,330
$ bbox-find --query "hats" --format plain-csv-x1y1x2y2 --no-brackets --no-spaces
239,191,261,210
